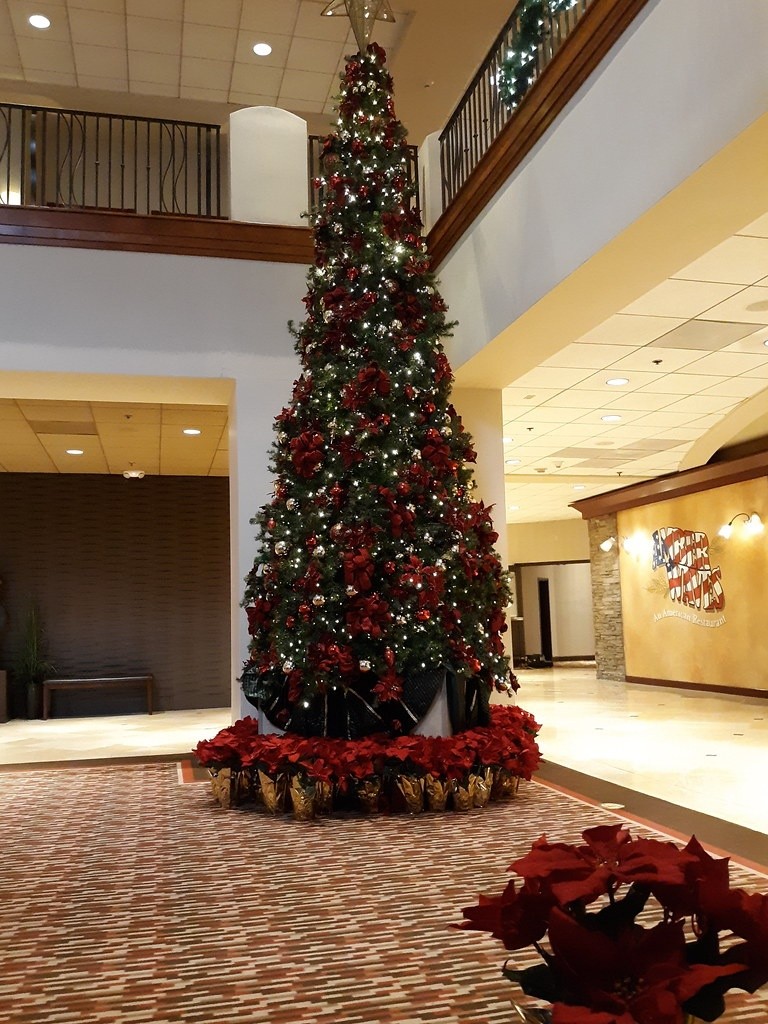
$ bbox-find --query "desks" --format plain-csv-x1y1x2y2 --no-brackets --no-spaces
41,675,157,722
